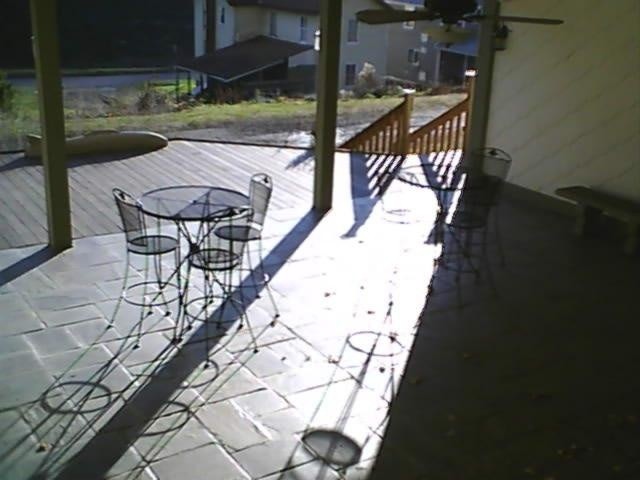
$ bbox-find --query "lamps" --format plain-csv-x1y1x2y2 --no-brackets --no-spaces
419,10,476,49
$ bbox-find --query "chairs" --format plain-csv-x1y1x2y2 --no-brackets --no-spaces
376,145,512,309
109,170,274,369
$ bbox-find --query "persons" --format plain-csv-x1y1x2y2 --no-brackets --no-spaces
191,80,201,96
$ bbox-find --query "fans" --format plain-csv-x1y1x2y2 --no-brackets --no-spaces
356,0,566,26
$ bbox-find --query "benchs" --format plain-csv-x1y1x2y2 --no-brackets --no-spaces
554,186,640,257
